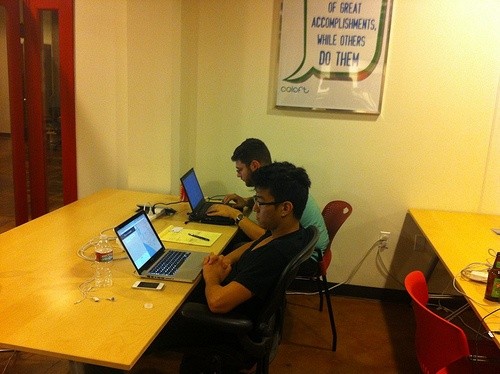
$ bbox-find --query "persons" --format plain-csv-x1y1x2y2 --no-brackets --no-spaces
84,161,314,374
205,138,330,276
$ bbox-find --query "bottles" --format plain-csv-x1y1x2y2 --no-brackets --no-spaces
484,251,500,302
95,235,113,287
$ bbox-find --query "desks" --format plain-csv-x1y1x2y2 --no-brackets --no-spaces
408,209,500,348
0,187,250,374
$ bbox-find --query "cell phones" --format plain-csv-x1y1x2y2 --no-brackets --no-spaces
131,281,164,291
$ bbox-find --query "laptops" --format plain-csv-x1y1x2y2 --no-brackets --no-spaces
180,167,243,225
113,210,213,283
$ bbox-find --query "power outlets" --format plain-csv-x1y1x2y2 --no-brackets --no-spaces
380,231,390,249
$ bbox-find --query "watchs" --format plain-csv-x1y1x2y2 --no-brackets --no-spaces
236,214,243,223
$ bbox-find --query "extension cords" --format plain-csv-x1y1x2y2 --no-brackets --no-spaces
146,208,165,221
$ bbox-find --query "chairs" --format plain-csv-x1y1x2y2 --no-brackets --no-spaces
404,270,500,374
165,225,319,374
288,200,353,351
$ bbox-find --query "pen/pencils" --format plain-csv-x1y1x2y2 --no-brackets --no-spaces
188,232,210,241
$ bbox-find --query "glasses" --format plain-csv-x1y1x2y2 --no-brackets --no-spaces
236,170,241,175
254,195,276,208
